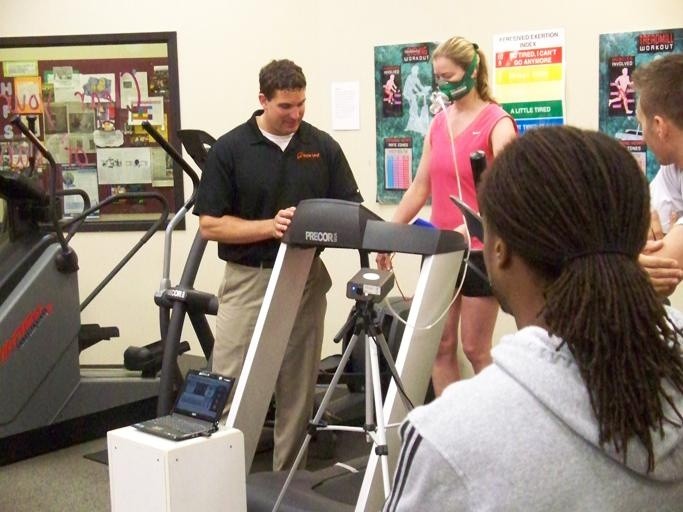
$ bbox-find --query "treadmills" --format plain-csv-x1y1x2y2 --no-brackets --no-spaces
225,196,465,512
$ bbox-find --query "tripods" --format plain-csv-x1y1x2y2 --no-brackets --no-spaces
272,299,414,512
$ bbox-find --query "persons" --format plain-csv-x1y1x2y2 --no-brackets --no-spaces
630,53,682,306
192,60,363,473
384,63,428,118
382,126,682,511
608,67,634,115
374,39,519,399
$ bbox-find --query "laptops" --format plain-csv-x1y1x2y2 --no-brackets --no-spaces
132,369,235,441
